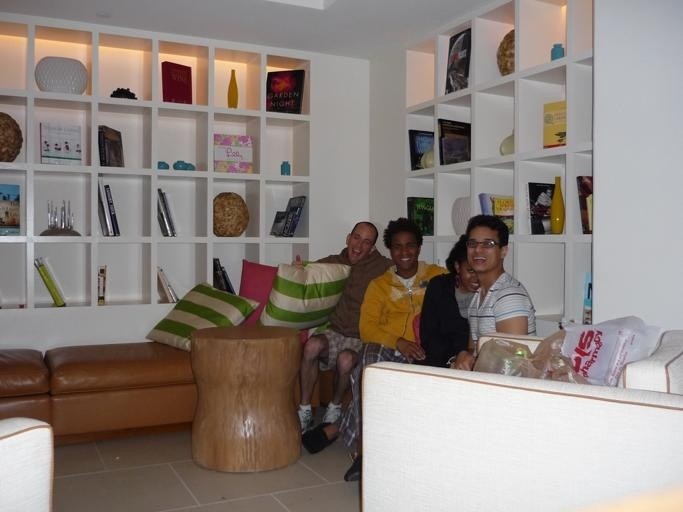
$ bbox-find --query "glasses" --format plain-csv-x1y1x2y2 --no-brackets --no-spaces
467,238,498,249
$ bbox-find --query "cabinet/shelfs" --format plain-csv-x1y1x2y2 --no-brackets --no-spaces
0,8,313,310
363,0,593,338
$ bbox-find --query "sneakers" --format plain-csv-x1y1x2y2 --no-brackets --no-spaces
298,404,340,454
343,452,362,482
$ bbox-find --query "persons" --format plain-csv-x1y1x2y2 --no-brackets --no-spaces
301,217,452,455
343,235,482,483
289,221,396,437
452,213,536,372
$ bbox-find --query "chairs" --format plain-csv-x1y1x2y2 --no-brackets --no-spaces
0,414,56,510
357,327,683,512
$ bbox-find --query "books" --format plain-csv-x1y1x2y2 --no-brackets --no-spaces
160,61,192,105
575,175,592,234
435,118,472,165
35,118,83,166
160,269,179,303
527,182,556,234
103,184,120,237
220,266,237,296
444,28,470,95
32,258,65,307
154,265,173,304
160,191,178,237
97,264,105,306
156,187,173,238
284,196,306,237
39,256,68,306
0,182,21,237
440,137,471,165
96,176,114,236
211,257,227,292
264,68,305,114
581,271,592,325
406,128,433,170
542,100,566,150
96,123,125,168
407,197,433,236
476,193,513,234
213,133,254,174
270,210,296,238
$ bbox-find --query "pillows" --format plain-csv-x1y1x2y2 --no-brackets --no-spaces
259,261,353,333
238,258,276,324
146,282,255,355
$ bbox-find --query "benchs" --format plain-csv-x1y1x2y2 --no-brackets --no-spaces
1,350,46,420
46,344,342,436
